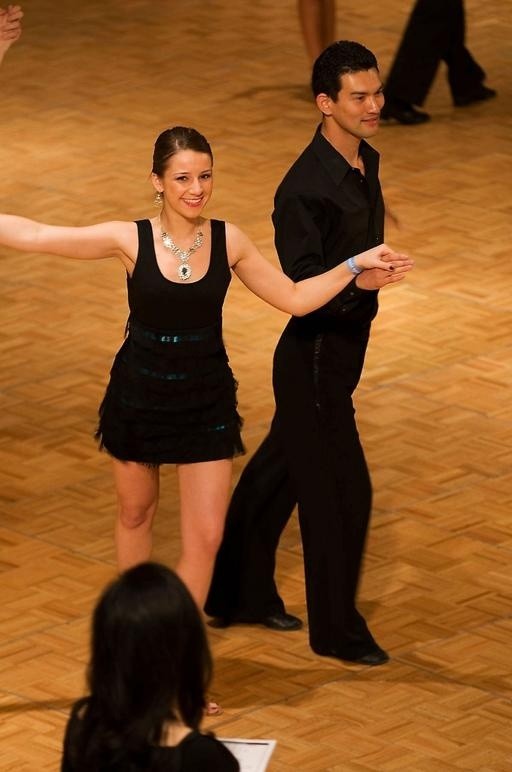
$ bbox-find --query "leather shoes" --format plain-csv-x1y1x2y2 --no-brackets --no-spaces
310,629,388,666
453,86,497,106
204,593,302,631
379,104,431,125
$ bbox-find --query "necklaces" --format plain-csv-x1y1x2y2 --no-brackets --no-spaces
153,221,207,281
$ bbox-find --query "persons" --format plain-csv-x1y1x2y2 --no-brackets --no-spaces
60,561,245,769
378,1,496,124
200,39,412,665
2,127,400,713
294,1,336,60
0,1,27,64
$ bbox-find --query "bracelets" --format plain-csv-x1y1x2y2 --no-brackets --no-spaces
343,256,363,276
